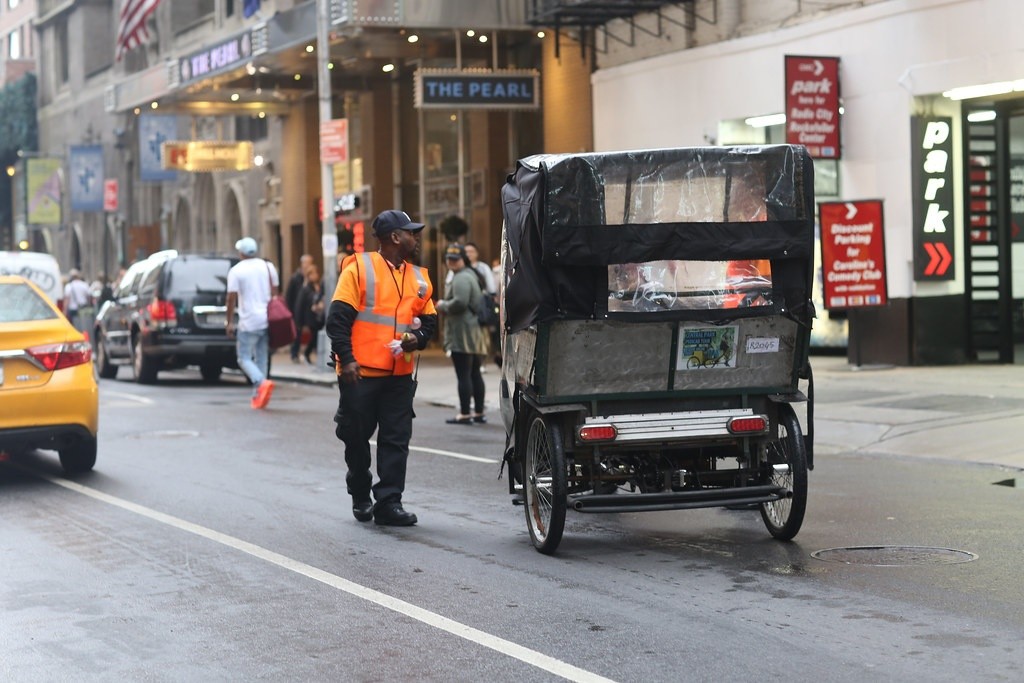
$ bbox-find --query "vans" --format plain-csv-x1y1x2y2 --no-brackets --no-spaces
0,250,64,311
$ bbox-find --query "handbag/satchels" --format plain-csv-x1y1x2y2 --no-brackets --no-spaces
266,261,296,350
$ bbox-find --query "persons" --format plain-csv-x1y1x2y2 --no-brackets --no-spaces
443,243,496,373
325,209,438,526
287,253,314,346
289,264,324,366
226,237,281,408
58,262,134,350
434,243,488,425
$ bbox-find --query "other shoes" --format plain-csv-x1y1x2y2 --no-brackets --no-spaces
446,417,474,424
471,416,488,423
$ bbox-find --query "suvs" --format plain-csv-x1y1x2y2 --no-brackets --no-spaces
92,249,270,387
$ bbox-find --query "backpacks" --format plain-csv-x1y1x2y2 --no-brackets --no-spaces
466,266,500,327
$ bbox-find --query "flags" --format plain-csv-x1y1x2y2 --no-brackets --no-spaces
117,0,161,59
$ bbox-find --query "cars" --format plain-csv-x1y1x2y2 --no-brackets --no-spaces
1,273,101,476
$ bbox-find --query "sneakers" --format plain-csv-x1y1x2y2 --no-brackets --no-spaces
257,381,275,408
252,388,261,407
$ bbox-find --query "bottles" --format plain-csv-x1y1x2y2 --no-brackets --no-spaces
409,316,422,330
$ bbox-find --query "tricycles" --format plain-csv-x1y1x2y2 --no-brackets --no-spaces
497,144,815,554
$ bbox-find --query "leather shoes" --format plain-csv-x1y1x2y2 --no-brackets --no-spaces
352,494,373,522
373,505,418,526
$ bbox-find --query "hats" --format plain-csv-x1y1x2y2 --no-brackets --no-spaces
445,245,466,260
371,210,425,238
235,236,258,257
